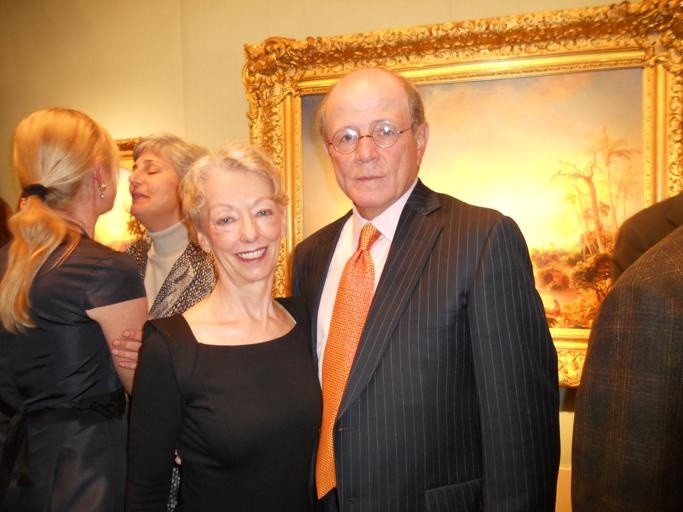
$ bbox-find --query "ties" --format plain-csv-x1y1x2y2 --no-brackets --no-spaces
316,224,380,503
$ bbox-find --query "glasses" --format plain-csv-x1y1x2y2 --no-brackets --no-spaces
328,122,412,156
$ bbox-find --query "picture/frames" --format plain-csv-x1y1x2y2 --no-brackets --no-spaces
242,0,683,393
92,138,151,253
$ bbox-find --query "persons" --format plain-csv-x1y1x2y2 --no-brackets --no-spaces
287,64,566,512
606,191,683,284
0,105,149,512
109,128,217,375
568,223,682,507
122,143,324,512
